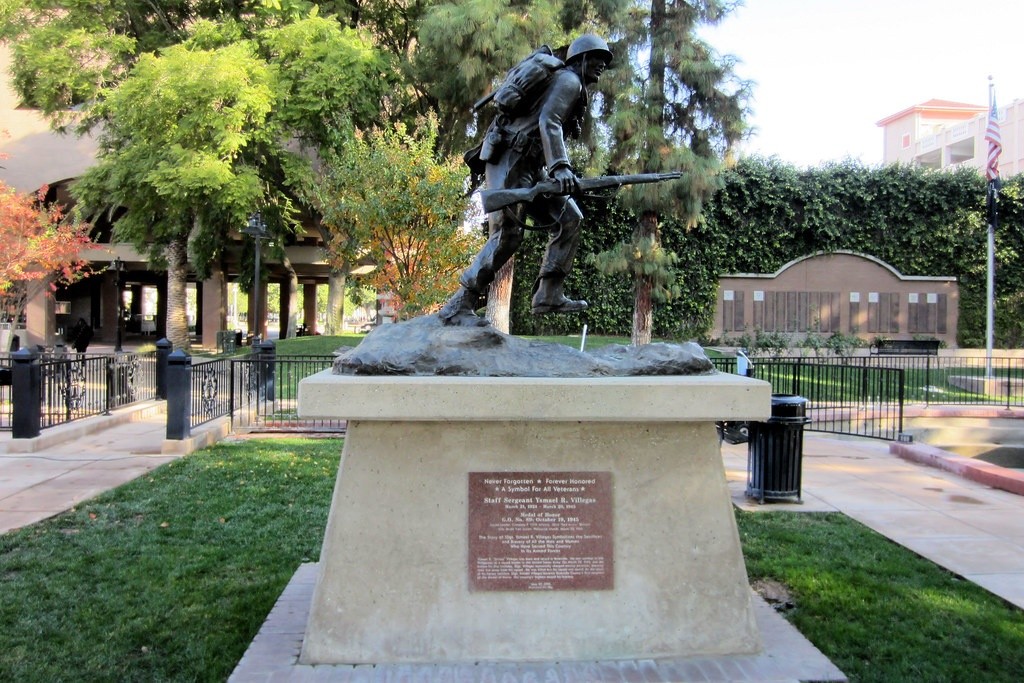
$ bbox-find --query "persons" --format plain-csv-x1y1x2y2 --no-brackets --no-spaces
439,35,613,317
71,318,91,365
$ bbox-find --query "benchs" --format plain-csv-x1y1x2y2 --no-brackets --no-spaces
869,339,941,366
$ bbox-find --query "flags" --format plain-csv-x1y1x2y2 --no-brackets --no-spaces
985,88,1002,182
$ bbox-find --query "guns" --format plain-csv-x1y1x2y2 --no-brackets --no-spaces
480,170,684,213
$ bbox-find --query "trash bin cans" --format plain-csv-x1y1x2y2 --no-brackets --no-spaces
746,393,813,504
106,351,138,408
